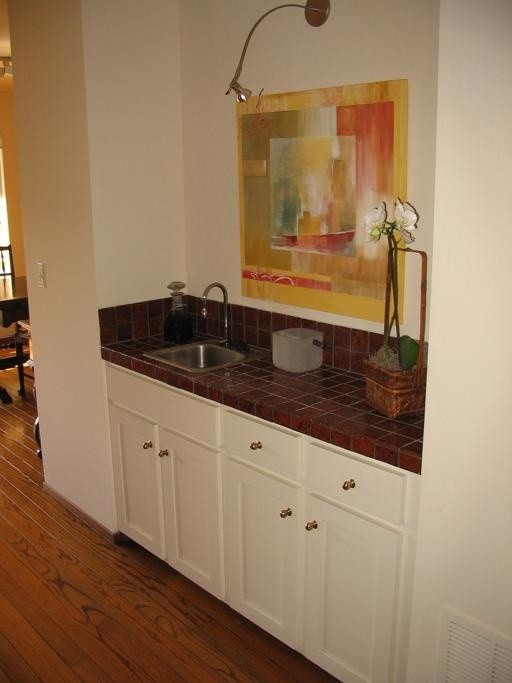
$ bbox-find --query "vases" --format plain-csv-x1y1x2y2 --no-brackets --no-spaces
365,357,426,418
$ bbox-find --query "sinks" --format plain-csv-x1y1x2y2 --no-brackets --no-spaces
143,340,248,375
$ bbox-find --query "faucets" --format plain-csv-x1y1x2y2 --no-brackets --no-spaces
202,282,231,345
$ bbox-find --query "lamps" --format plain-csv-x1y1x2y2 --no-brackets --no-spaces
225,0,330,103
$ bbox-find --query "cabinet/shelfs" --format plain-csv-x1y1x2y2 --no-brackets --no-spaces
105,362,224,603
224,405,420,683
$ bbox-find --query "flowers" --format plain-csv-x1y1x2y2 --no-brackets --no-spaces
363,197,419,370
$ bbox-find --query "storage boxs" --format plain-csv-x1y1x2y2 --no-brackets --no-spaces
272,328,323,373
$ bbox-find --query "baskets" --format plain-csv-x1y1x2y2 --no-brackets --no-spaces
365,246,426,419
